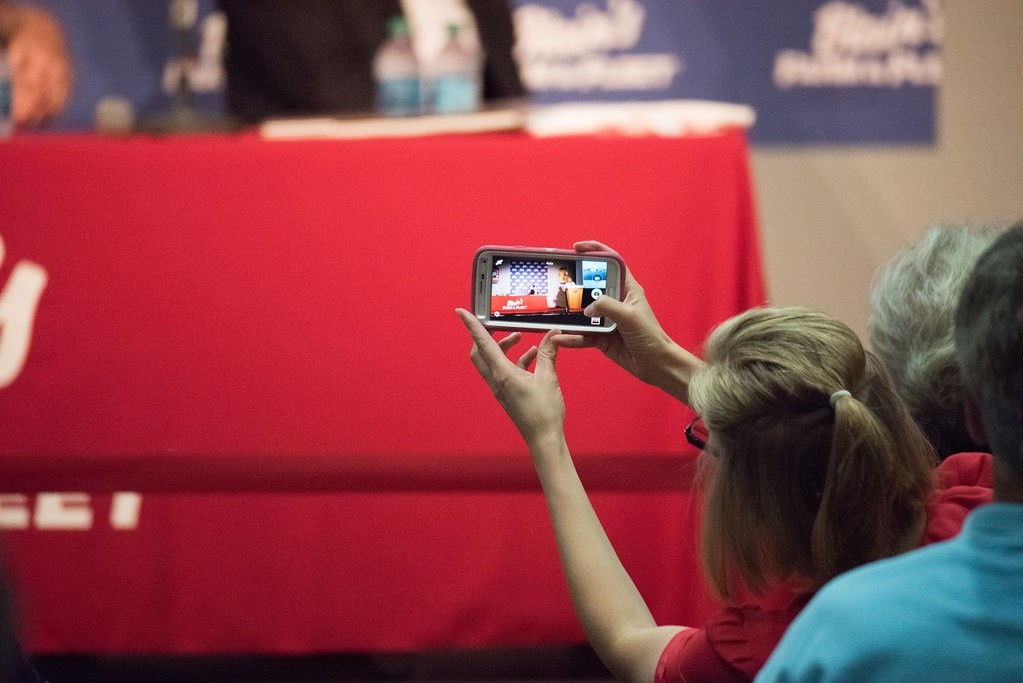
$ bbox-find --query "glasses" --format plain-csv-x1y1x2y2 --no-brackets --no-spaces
685,415,726,464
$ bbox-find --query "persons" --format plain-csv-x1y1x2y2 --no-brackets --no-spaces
529,283,539,294
753,219,1022,683
217,0,531,128
866,221,994,456
562,277,578,313
455,217,1021,683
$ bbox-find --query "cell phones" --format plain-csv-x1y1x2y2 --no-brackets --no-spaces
472,244,626,337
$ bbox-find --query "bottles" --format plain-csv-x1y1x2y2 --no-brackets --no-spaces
433,23,479,113
371,16,422,116
